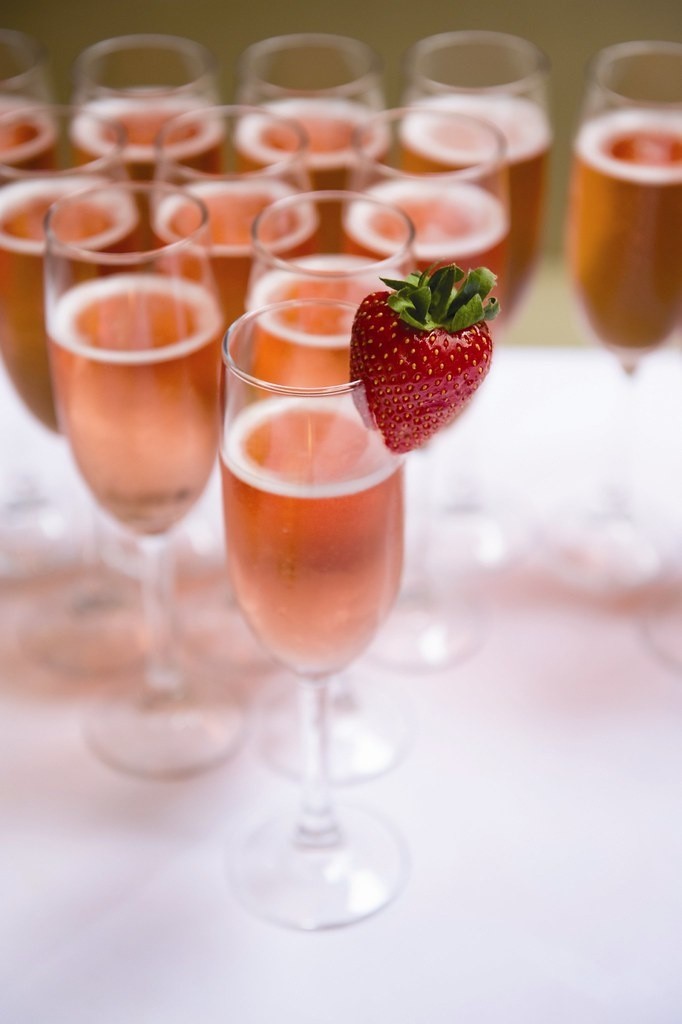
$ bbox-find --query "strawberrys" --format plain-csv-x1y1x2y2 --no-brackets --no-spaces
347,257,501,455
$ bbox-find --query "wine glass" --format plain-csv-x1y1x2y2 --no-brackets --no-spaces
564,38,682,584
221,301,405,934
2,29,551,649
252,193,410,782
46,183,233,770
